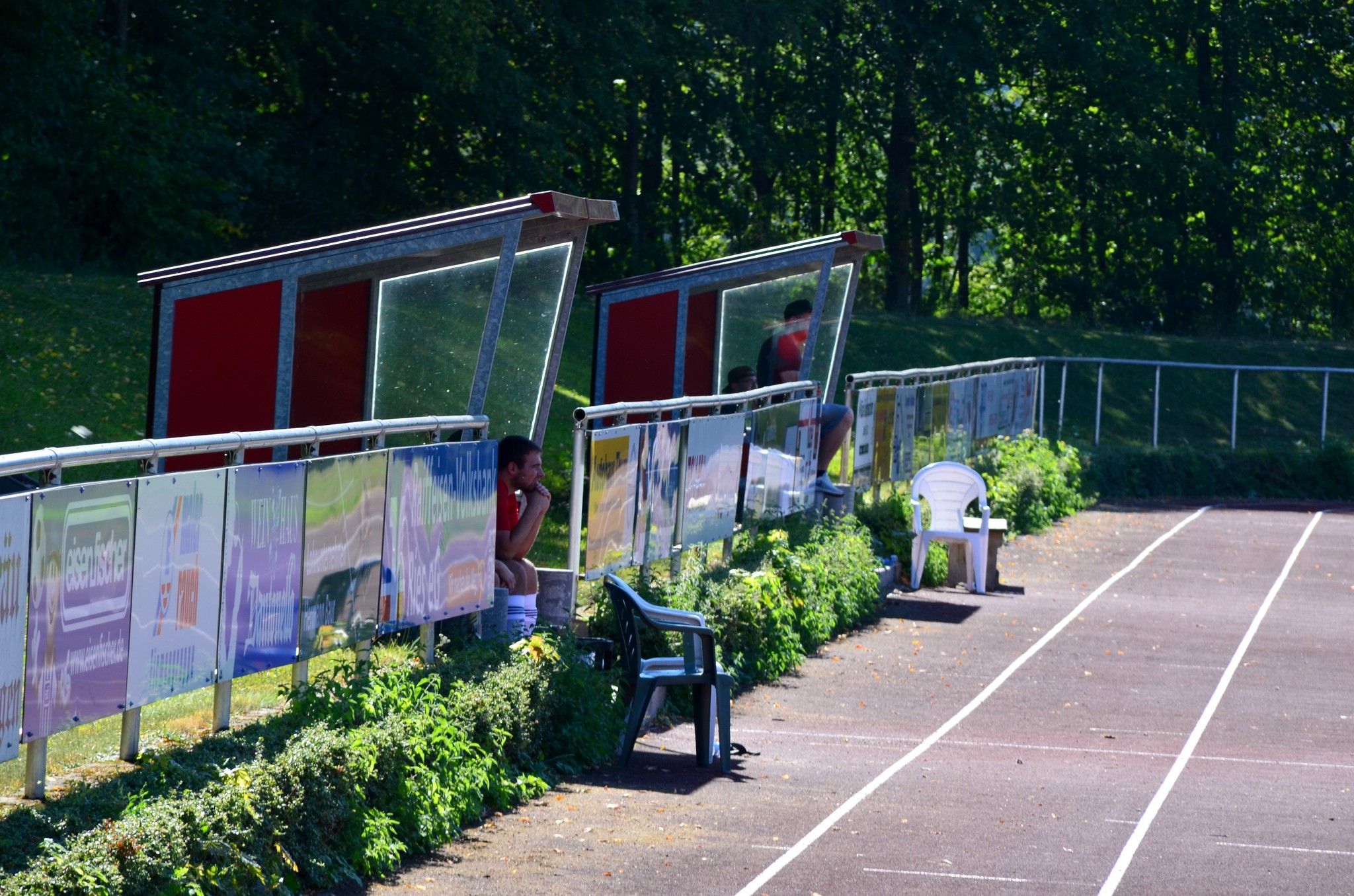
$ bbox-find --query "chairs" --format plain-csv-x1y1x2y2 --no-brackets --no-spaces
909,460,991,594
603,572,733,777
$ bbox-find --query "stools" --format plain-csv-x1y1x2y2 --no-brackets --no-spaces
949,514,1008,588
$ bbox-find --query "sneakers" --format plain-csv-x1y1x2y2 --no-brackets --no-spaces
816,472,845,496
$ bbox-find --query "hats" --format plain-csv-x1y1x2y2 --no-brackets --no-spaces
723,366,755,394
784,300,824,320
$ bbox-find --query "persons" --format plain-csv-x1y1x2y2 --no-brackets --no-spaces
758,300,853,497
443,430,551,640
721,366,758,411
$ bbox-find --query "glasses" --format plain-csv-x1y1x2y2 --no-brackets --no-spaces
736,379,758,388
